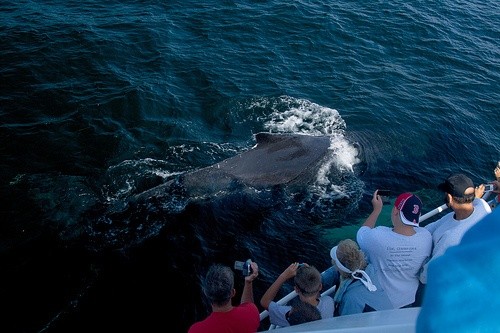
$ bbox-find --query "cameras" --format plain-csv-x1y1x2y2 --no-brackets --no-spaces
484,184,493,192
376,190,391,204
234,259,252,276
297,264,304,269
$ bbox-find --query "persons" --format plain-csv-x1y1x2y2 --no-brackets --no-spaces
330,239,368,315
190,262,260,333
261,262,334,328
420,174,492,285
474,166,500,198
356,189,432,311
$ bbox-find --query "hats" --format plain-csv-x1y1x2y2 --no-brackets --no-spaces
438,173,475,199
395,192,423,227
330,244,377,292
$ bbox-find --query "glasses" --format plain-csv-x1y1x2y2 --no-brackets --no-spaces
285,309,292,326
